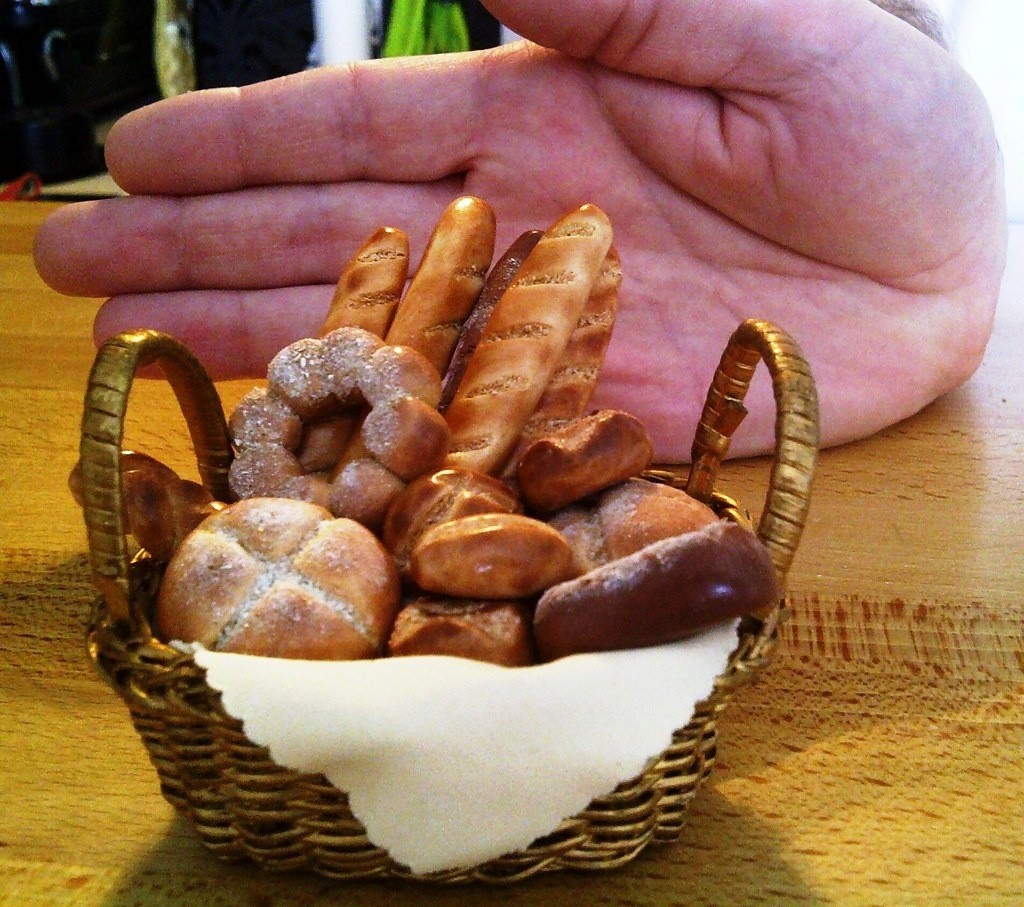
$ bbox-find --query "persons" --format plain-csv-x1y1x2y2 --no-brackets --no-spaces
32,0,1006,465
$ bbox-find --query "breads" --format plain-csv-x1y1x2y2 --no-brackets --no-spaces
162,191,778,658
